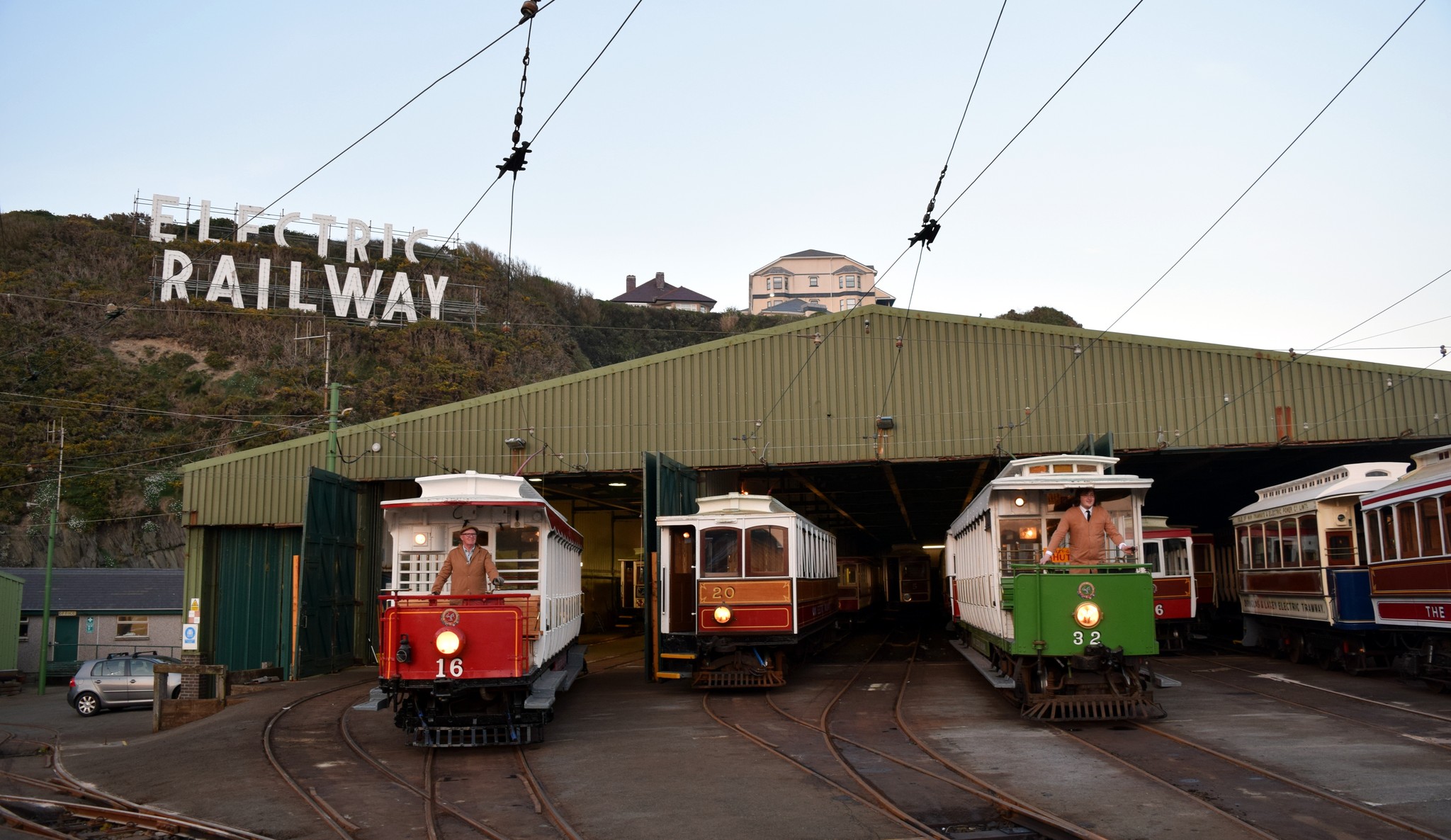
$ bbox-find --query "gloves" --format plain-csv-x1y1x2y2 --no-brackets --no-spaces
494,576,504,586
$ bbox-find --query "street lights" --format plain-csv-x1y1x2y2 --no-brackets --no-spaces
326,383,355,474
25,501,57,696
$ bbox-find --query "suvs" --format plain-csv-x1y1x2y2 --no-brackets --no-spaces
67,650,183,717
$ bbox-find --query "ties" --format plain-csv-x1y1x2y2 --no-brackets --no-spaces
1086,510,1091,522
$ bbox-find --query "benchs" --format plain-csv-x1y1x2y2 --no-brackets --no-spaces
398,594,545,641
999,576,1015,611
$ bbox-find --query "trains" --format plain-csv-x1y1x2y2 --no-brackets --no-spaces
617,559,646,628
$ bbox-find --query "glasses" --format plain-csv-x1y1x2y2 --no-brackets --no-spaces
1080,494,1094,498
461,533,477,537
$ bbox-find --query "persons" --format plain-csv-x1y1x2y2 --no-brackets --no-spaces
427,524,504,606
1040,488,1137,574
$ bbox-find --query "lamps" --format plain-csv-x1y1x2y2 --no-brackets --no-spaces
877,416,895,430
504,438,527,450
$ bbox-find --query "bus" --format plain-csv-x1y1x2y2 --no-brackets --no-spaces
378,470,590,747
654,492,933,688
1227,443,1451,695
939,452,1222,728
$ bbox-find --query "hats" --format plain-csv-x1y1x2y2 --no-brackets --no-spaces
459,526,479,533
